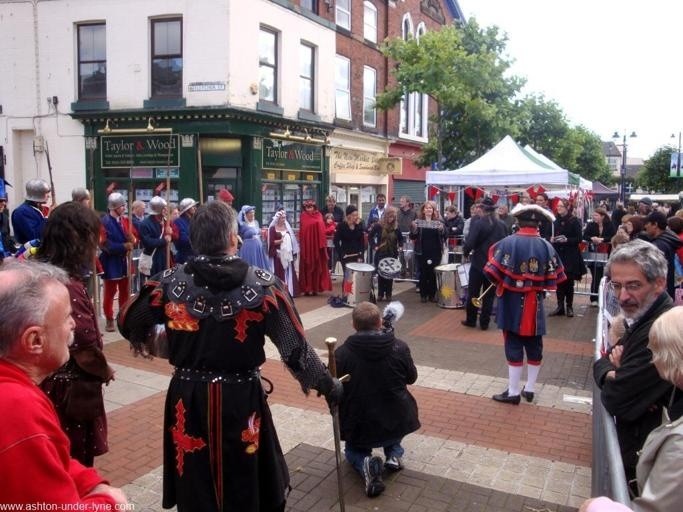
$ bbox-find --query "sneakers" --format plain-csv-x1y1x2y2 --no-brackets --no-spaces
421,296,426,303
105,319,115,332
384,457,404,470
429,296,437,303
363,456,385,498
591,301,598,307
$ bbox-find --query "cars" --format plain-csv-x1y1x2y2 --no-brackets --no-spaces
609,186,634,196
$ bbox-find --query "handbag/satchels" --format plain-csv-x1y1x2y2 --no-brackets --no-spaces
137,251,153,276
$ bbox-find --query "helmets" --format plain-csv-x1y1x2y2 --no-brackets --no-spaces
72,187,89,202
148,196,167,215
24,178,50,203
106,193,126,210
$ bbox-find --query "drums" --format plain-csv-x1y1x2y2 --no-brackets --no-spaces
447,251,472,283
342,263,376,307
376,257,402,281
434,263,468,309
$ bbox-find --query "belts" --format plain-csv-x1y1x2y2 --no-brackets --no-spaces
172,366,260,382
55,368,79,380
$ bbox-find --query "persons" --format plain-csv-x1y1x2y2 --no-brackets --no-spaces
320,300,422,498
120,197,343,511
0,255,132,511
0,167,683,333
482,200,569,409
574,303,682,512
33,197,117,474
589,236,683,499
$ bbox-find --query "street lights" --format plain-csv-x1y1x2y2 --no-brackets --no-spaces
612,128,637,208
670,132,682,194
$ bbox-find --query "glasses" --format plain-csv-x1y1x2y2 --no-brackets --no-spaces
609,282,642,291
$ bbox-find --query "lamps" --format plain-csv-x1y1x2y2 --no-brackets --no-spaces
320,131,331,144
285,125,290,137
304,128,311,142
104,118,118,135
147,117,159,132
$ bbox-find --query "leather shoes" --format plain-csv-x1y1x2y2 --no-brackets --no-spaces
377,296,383,302
386,294,391,301
567,308,574,317
521,385,534,402
492,389,520,405
548,308,565,316
462,320,476,327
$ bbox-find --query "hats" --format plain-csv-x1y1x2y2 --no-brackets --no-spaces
0,179,7,200
509,202,557,224
667,217,682,234
345,205,357,216
239,205,256,221
480,197,499,209
179,198,199,216
639,198,652,206
